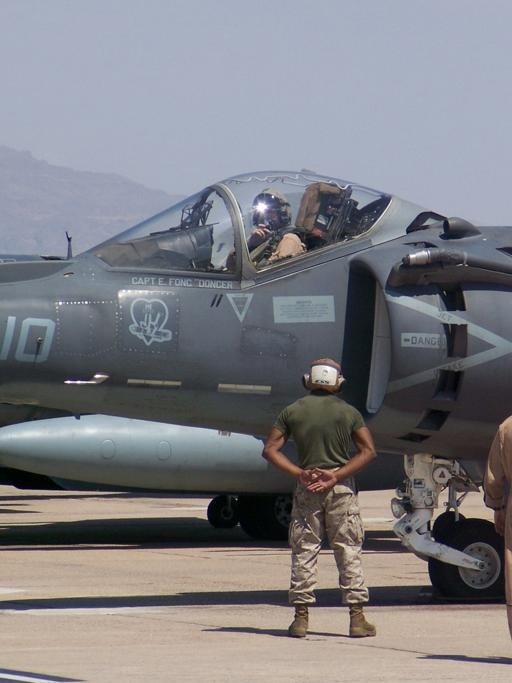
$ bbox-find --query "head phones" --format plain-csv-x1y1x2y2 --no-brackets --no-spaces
302,369,347,392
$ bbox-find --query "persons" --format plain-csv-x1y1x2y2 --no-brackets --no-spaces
225,184,306,273
261,357,376,638
481,413,512,642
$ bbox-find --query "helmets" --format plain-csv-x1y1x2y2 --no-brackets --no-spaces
301,356,346,397
250,186,291,227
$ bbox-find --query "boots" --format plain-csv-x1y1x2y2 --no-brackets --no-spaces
348,602,377,638
287,603,310,637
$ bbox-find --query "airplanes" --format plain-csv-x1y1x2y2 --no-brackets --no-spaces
0,166,511,607
